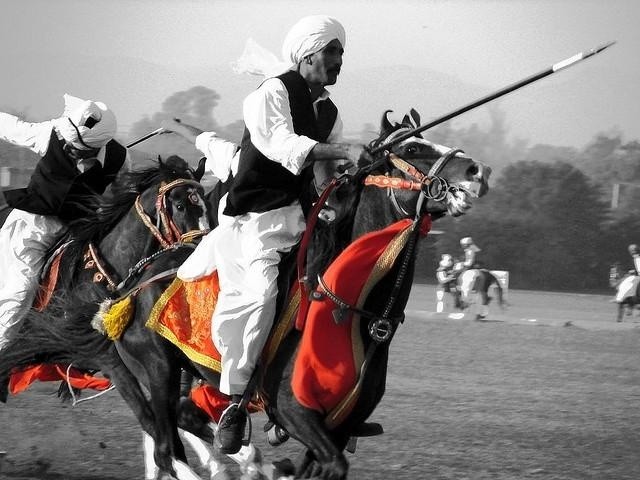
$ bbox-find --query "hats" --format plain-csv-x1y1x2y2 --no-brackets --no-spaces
460,237,473,245
283,14,346,63
58,100,117,151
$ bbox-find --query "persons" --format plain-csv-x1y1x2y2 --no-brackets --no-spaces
613,243,640,304
160,116,241,234
458,236,483,303
211,11,376,456
434,253,456,314
1,94,132,356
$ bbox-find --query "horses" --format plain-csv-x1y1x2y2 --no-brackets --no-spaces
446,258,510,320
0,107,491,480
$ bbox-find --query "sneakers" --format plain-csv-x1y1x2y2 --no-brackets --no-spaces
214,402,247,454
